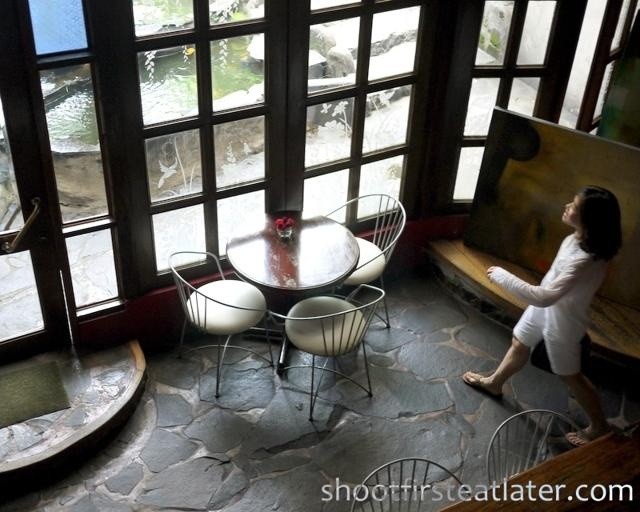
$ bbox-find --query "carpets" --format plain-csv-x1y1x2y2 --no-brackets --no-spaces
0,359,73,432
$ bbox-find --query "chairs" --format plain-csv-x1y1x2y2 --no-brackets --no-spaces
343,406,640,512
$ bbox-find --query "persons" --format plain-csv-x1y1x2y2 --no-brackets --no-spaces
460,185,625,447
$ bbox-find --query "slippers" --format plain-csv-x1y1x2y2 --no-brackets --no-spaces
463,370,504,401
565,429,598,447
